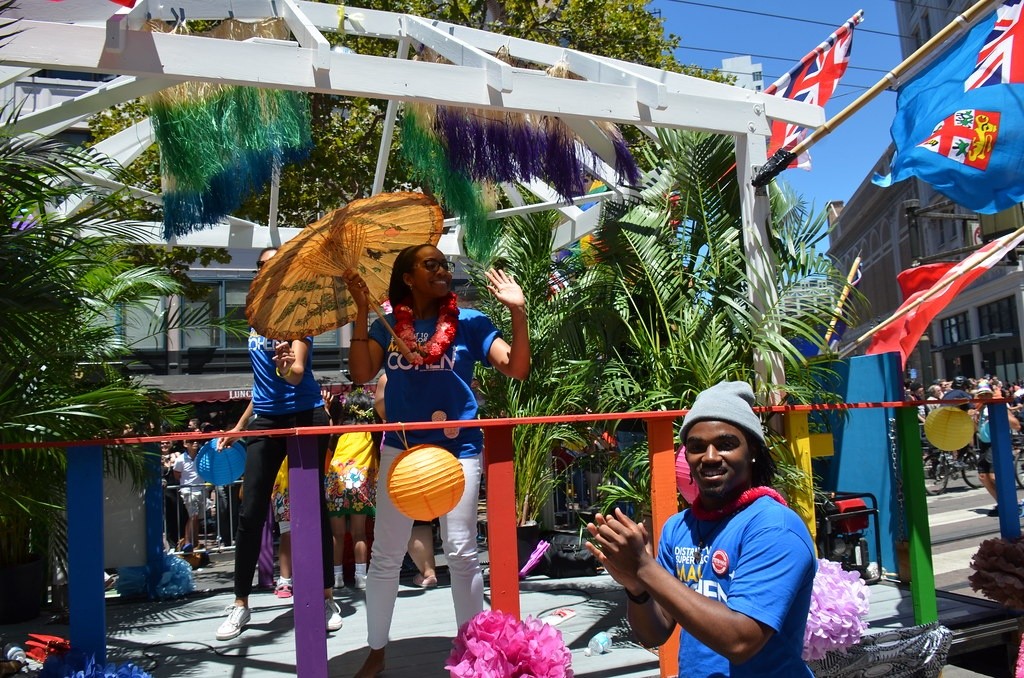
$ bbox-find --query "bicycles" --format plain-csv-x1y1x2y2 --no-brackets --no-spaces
922,432,1024,497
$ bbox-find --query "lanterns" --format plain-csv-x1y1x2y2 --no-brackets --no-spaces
924,406,974,451
672,445,700,505
194,437,247,487
386,445,465,522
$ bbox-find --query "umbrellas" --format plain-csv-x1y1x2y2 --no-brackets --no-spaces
245,192,443,364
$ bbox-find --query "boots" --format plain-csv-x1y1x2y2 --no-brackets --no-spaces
353,647,386,678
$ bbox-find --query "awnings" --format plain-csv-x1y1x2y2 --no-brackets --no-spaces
138,370,352,405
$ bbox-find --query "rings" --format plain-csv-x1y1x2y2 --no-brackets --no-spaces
358,282,362,288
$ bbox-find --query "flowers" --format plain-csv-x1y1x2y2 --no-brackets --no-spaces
802,558,871,661
339,386,374,419
968,537,1024,612
155,552,195,601
37,646,156,678
444,610,575,678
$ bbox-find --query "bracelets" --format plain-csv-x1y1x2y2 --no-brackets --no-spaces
624,587,652,605
350,339,368,341
276,367,292,378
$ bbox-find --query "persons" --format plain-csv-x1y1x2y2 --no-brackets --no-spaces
905,374,1024,518
161,407,436,599
328,242,531,678
214,247,343,641
585,381,819,678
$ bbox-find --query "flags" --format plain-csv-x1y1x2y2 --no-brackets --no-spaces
765,13,864,171
866,227,1024,372
870,0,1024,215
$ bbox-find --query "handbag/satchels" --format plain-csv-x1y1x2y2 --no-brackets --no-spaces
25,631,71,665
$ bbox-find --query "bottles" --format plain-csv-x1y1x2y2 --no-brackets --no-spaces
4,641,26,666
584,631,612,657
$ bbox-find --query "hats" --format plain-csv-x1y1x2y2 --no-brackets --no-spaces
679,380,766,446
910,383,921,391
976,379,994,396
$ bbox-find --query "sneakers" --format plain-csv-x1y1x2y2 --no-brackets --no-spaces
324,598,343,630
988,502,999,515
334,576,344,588
1018,499,1024,517
215,603,251,640
413,573,437,587
273,576,293,597
354,572,367,588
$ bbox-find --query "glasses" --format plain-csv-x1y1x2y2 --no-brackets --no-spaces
409,256,456,274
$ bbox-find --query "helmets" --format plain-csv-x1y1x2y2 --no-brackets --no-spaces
951,376,971,389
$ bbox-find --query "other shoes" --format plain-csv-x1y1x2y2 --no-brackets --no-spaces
251,583,274,593
182,543,192,558
